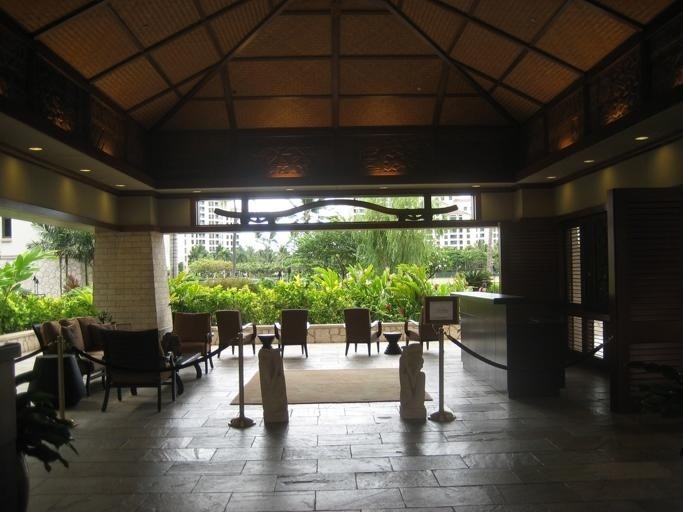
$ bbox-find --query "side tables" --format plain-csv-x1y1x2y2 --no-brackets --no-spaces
27,354,84,410
257,334,276,357
383,332,403,354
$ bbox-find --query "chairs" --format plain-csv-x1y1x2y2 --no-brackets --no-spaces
275,309,310,359
100,327,176,412
33,316,114,397
344,308,382,356
405,306,440,355
173,312,213,374
216,310,257,359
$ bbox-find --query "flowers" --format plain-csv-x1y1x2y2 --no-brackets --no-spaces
161,332,181,352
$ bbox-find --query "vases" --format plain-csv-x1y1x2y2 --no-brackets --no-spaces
167,345,177,361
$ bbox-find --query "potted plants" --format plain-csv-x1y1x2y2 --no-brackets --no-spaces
16,390,80,512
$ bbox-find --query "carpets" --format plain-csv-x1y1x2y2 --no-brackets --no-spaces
230,368,432,405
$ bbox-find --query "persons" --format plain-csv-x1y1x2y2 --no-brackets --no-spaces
479,283,487,292
